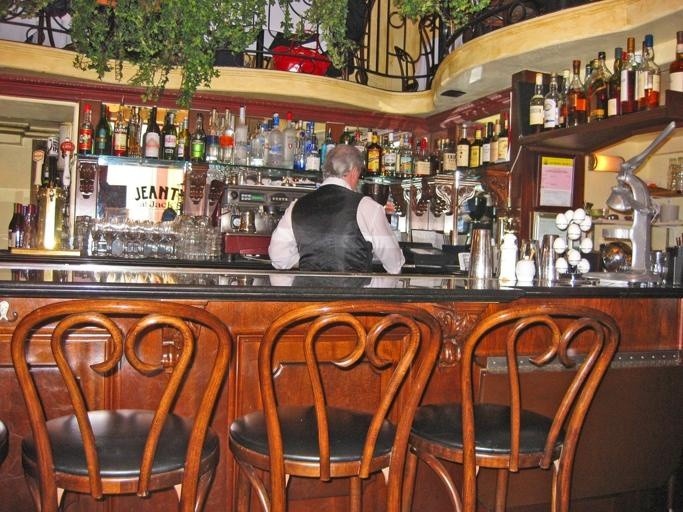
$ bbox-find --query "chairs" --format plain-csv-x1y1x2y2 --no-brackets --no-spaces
418,11,442,91
394,45,419,92
228,301,443,512
10,299,232,511
402,302,620,511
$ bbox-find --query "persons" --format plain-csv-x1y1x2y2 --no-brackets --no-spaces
267,143,408,276
268,274,398,289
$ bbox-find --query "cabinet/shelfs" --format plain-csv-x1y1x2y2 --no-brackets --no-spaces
73,81,511,276
511,59,683,273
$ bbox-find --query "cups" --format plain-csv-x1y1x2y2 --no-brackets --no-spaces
553,207,592,275
516,232,559,282
660,204,677,222
463,253,469,272
221,170,264,186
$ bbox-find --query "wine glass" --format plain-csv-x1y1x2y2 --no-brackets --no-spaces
75,208,221,257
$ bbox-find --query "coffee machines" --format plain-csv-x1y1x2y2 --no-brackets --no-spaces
217,184,317,257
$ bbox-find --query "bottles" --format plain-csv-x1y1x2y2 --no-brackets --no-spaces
669,156,683,193
528,32,683,135
6,203,37,249
468,222,493,279
37,134,63,249
79,103,510,178
498,221,519,281
